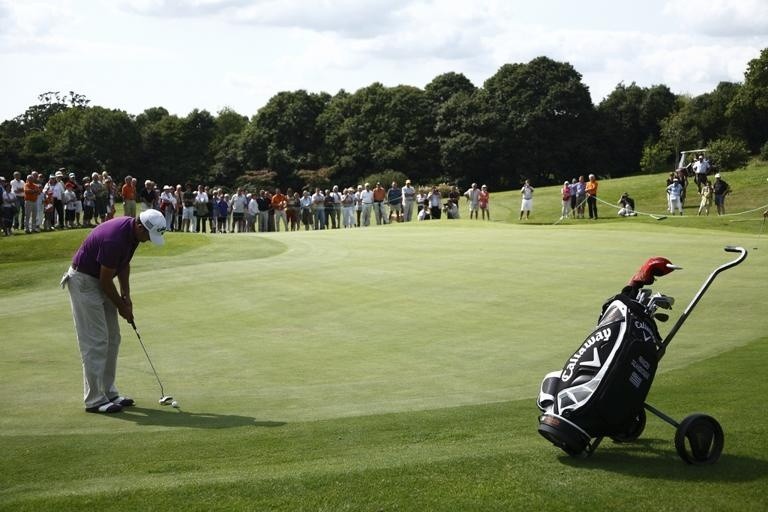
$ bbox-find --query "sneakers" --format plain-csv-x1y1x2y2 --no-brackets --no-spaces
84,402,122,414
110,395,134,409
1,221,98,238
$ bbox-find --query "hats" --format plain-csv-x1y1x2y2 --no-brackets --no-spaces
1,167,136,200
667,154,723,185
139,209,167,246
145,179,412,198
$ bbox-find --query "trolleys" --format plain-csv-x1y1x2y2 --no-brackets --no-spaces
538,240,748,471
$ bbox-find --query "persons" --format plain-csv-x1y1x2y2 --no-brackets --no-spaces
666,155,730,216
617,192,634,216
60,209,167,413
568,178,577,219
585,174,598,220
519,180,534,220
572,176,586,218
1,168,490,236
560,181,572,220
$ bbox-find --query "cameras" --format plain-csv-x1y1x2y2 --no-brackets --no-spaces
435,190,439,192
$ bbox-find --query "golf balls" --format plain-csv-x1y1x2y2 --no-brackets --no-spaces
172,399,178,406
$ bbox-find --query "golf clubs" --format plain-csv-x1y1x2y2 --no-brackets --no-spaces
120,295,173,404
637,288,675,323
753,217,765,249
